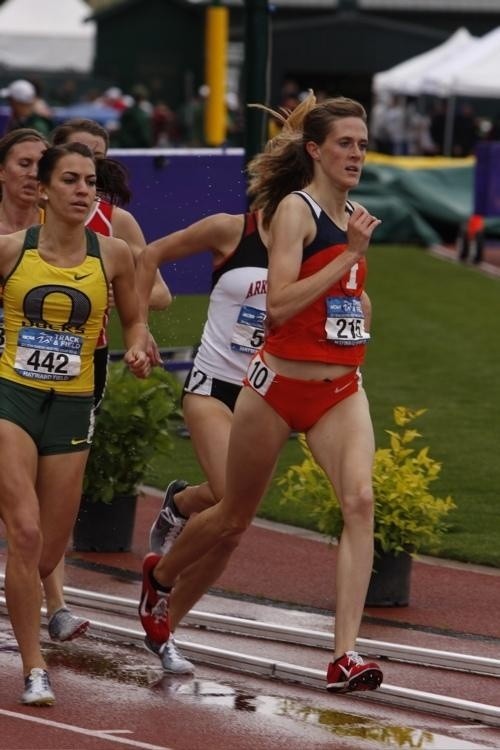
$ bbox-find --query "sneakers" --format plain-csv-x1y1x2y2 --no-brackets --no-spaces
144,631,196,674
19,667,57,706
149,479,192,558
47,606,90,643
326,650,383,694
138,552,176,644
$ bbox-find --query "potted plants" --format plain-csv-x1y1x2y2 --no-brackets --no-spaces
74,360,185,552
274,407,456,608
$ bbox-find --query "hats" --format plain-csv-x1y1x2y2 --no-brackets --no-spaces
0,79,37,104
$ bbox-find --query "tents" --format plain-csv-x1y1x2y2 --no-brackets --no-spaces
375,26,500,156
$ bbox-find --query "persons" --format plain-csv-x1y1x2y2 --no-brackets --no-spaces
0,75,172,431
136,93,385,696
0,140,162,709
154,70,500,265
132,132,315,679
